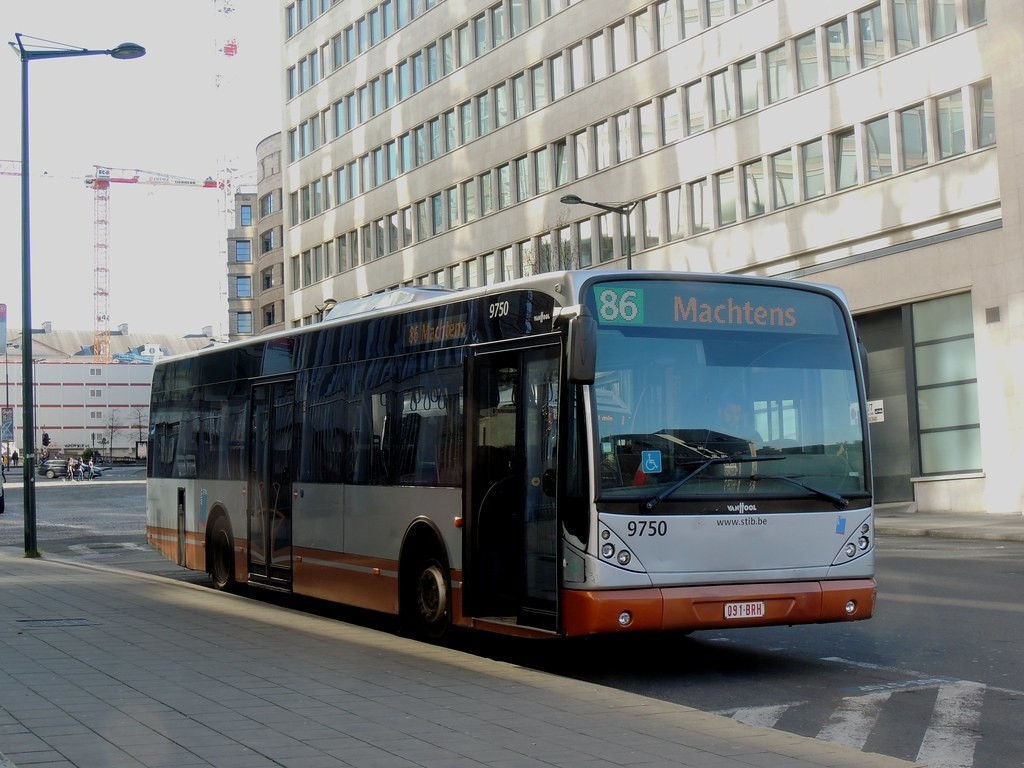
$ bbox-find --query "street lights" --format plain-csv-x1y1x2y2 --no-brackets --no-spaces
7,30,146,558
4,341,20,472
559,193,633,272
32,357,46,464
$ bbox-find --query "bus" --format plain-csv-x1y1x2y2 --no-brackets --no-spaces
144,268,880,641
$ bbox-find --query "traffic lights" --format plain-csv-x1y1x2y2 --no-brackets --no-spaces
42,434,51,446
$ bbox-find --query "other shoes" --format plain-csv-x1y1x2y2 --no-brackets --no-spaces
62,478,65,481
71,479,76,482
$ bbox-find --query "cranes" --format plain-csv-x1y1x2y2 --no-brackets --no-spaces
68,164,229,360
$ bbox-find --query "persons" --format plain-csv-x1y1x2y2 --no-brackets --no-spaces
88,457,95,481
693,393,765,453
39,446,62,463
76,457,85,481
61,458,76,482
2,453,7,467
12,450,19,466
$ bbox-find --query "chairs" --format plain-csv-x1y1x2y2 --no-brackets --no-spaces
148,412,462,486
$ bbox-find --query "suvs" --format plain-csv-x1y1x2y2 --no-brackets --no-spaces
38,460,103,479
